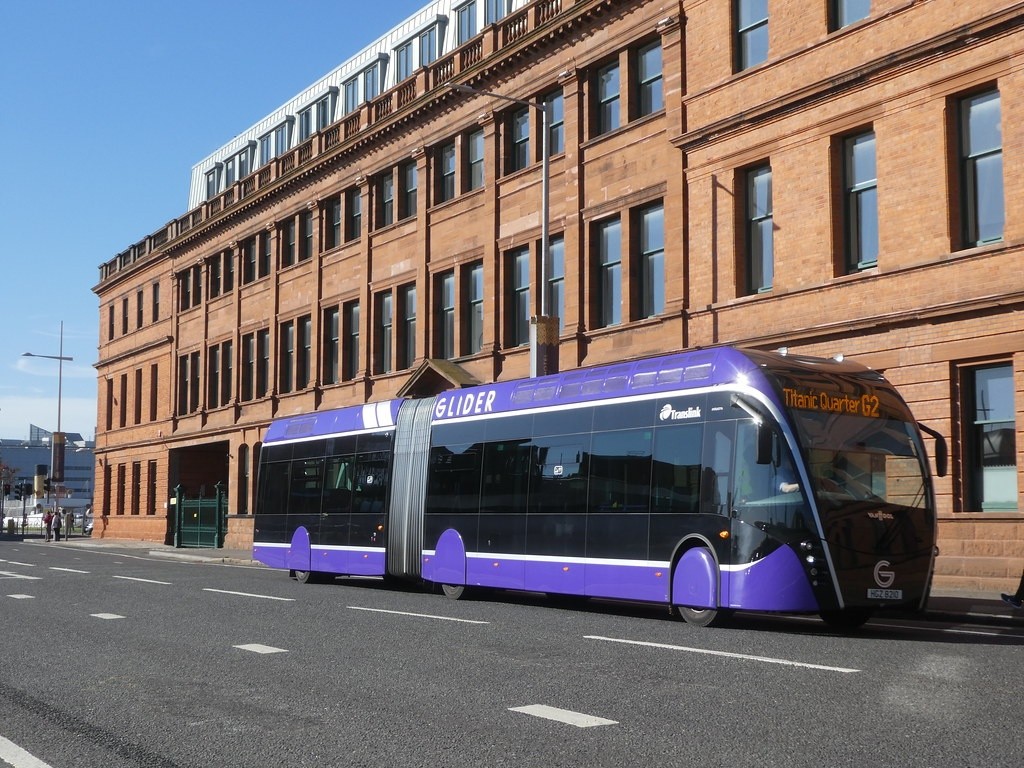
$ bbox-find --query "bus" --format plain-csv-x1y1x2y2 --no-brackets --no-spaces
250,344,949,628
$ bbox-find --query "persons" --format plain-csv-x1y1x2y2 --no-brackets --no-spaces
773,459,800,496
1000,568,1024,609
52,512,62,542
43,511,52,542
69,510,75,531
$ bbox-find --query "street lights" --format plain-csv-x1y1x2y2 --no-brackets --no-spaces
443,82,560,376
20,320,73,514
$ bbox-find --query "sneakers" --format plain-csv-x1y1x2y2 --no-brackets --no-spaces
1001,593,1022,608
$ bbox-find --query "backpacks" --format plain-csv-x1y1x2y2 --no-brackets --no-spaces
55,516,61,527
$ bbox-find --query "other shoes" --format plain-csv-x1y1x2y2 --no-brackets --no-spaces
46,540,50,542
